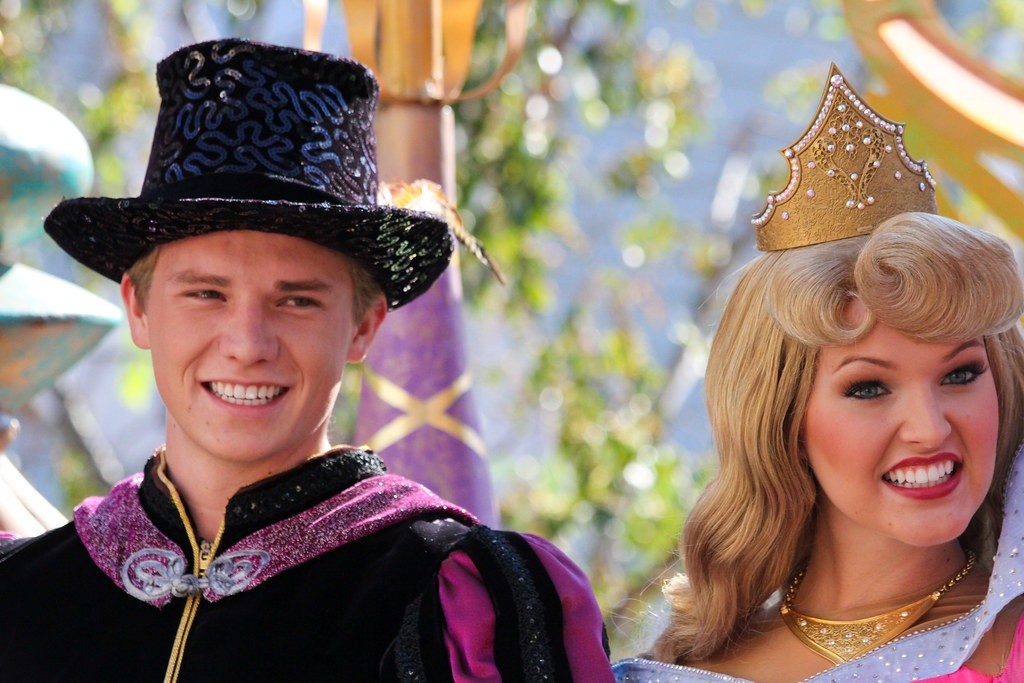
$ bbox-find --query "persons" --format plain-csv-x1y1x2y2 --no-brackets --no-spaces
0,36,620,683
611,210,1024,683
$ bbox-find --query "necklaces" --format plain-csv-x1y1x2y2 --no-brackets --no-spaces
779,544,976,666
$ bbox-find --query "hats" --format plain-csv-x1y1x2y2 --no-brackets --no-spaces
43,36,455,311
